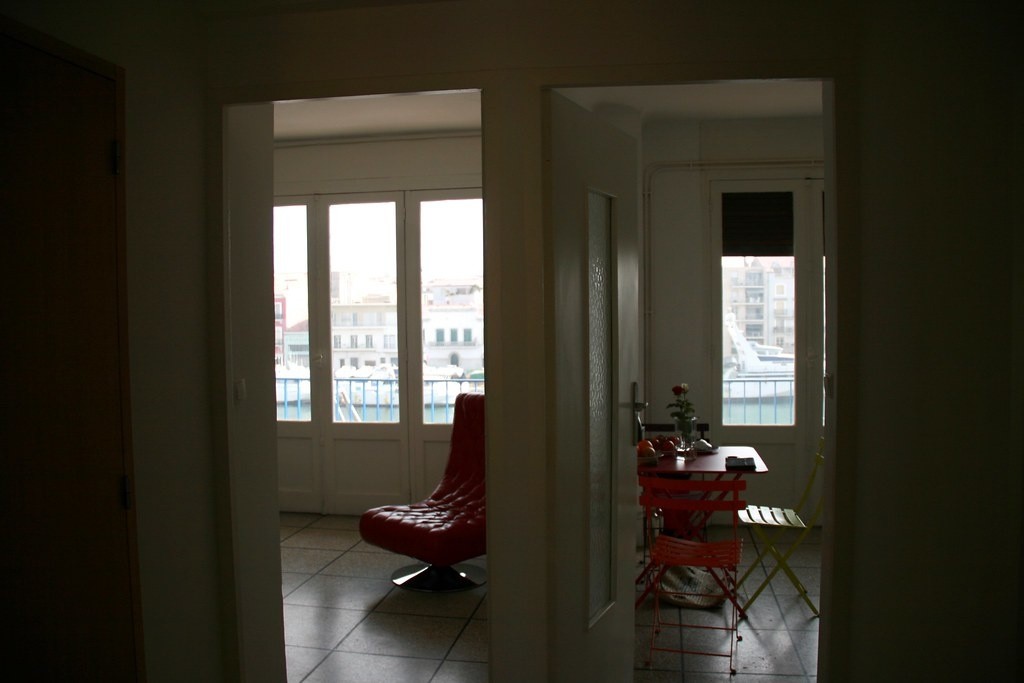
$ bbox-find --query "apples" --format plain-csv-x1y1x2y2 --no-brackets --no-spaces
638,434,679,456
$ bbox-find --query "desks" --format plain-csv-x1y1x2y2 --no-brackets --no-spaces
640,445,769,618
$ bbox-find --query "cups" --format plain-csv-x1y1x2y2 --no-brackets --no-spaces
684,434,697,461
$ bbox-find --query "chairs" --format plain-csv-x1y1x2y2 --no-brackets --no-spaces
358,392,485,592
737,424,825,617
639,475,748,677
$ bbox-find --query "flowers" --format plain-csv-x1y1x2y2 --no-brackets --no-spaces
665,383,697,442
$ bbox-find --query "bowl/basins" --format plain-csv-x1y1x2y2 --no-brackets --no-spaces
637,457,653,466
661,450,677,459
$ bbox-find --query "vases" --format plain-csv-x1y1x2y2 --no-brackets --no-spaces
675,417,697,461
